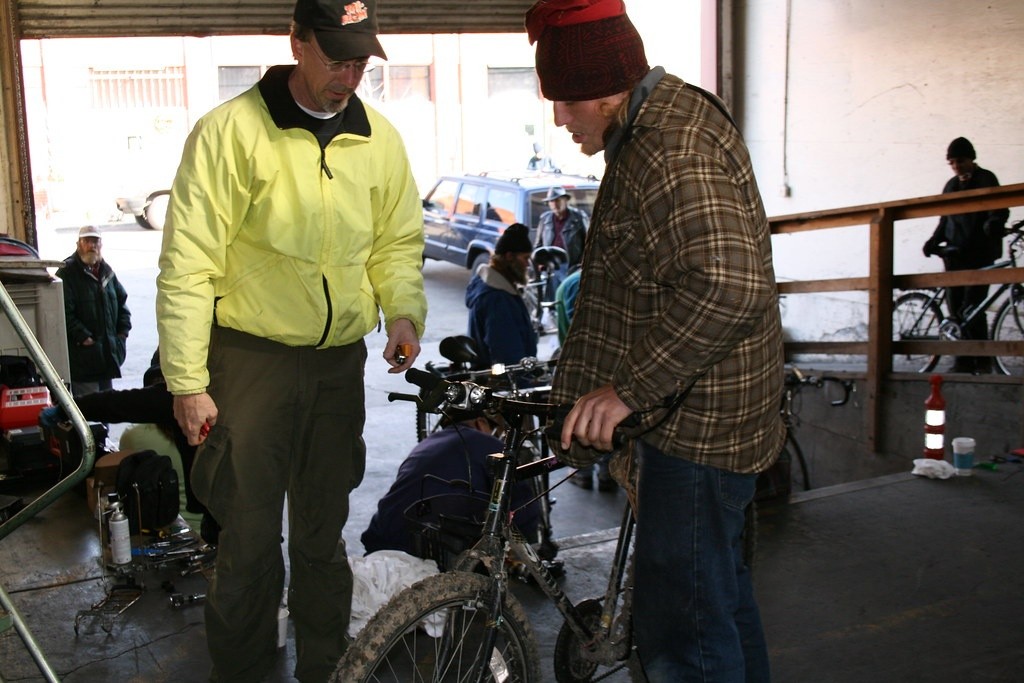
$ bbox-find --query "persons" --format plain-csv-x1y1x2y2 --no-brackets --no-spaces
465,222,538,451
154,0,428,683
523,0,789,683
531,186,589,306
923,138,1009,374
54,225,132,397
360,409,507,577
117,344,222,545
553,269,583,347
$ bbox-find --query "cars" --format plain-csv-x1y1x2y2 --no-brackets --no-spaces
114,167,178,231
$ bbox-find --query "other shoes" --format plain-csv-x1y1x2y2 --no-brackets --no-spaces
567,470,593,490
599,480,618,494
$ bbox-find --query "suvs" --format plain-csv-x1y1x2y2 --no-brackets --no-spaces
421,167,602,280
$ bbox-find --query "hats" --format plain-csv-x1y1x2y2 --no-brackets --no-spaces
78,225,102,237
440,408,501,439
540,187,571,203
946,137,977,158
293,0,388,61
524,0,650,101
495,224,532,253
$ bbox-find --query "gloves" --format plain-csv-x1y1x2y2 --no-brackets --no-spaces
39,403,69,432
984,219,1001,235
923,236,936,257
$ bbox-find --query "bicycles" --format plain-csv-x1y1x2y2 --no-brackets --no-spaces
781,360,850,491
326,334,648,683
893,219,1024,376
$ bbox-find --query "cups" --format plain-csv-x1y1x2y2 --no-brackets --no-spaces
952,437,977,476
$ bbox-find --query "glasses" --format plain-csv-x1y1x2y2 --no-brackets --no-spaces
307,41,379,75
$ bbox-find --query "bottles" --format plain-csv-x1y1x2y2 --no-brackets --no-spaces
109,502,132,564
105,493,123,548
924,373,946,460
